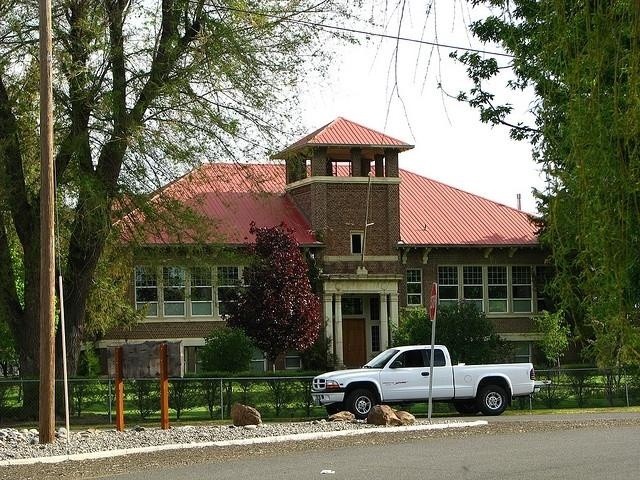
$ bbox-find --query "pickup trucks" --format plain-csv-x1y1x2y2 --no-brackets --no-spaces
310,344,534,416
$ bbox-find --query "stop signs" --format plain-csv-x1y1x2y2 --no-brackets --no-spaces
428,281,437,322
